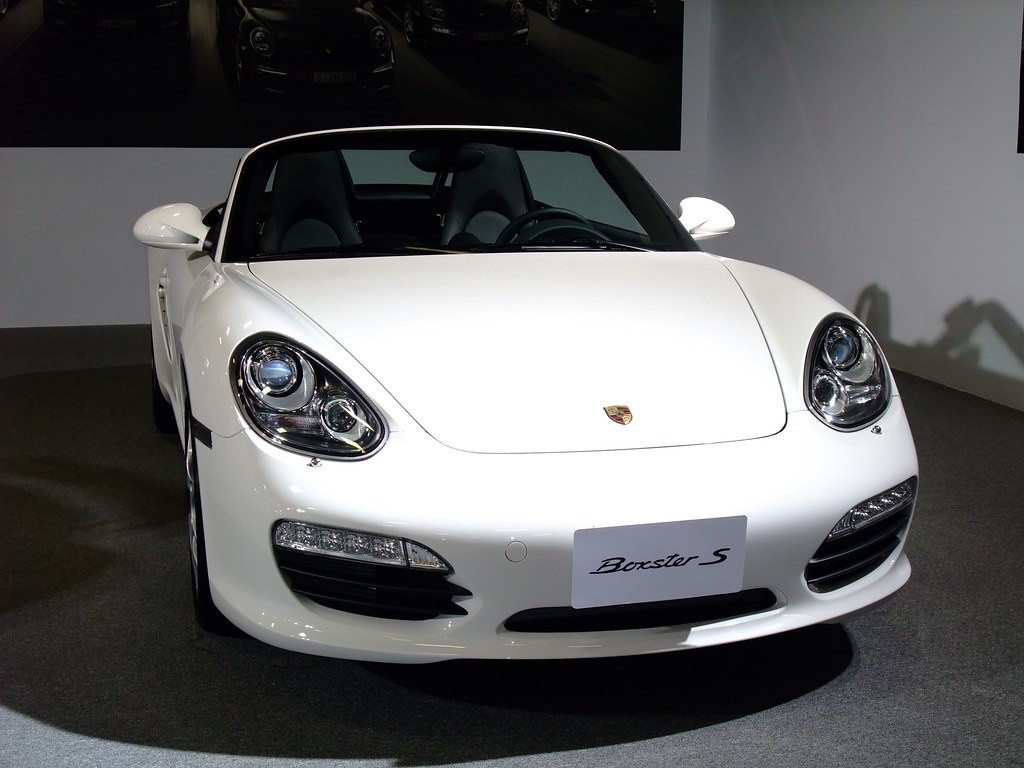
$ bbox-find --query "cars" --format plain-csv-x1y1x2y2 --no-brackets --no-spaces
2,0,657,96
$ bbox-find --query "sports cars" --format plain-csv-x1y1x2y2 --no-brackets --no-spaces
130,123,919,666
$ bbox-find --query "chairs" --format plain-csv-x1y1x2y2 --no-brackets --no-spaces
258,149,364,251
440,144,535,247
